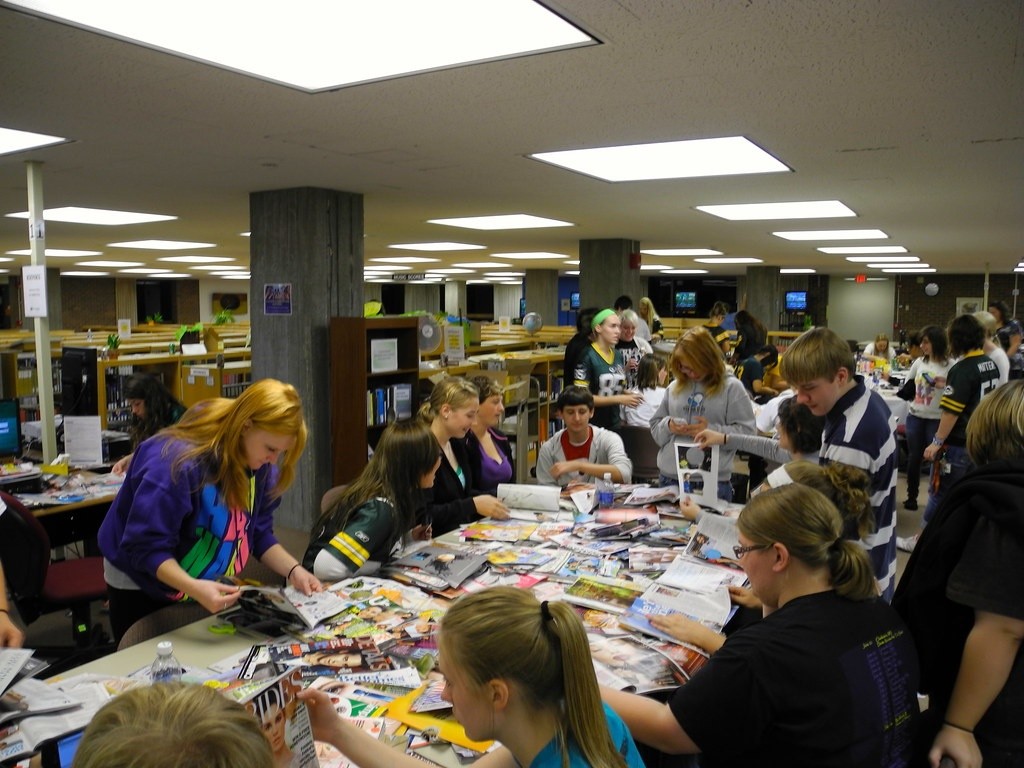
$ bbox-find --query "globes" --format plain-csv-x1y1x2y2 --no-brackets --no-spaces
523,312,543,338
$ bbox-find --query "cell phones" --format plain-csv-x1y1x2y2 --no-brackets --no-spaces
922,373,937,384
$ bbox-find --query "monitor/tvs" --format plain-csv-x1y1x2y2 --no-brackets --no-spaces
784,290,808,312
0,397,22,457
570,291,579,309
674,290,697,309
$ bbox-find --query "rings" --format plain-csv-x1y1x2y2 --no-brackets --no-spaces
224,603,226,609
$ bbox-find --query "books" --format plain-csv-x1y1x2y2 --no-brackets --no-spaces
107,368,251,422
0,444,753,768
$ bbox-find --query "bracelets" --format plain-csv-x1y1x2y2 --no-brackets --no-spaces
288,564,299,579
944,721,974,733
724,434,726,445
933,438,942,446
0,609,8,615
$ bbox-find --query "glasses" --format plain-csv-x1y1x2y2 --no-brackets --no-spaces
677,364,697,375
732,545,768,559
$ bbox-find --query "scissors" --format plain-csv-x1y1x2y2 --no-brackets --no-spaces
209,620,253,640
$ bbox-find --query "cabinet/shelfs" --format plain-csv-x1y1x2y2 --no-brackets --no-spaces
0,319,812,490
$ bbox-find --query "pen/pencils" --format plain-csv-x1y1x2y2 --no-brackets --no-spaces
501,496,506,502
391,548,399,556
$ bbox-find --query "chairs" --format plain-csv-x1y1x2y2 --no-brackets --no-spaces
0,484,111,679
894,425,936,474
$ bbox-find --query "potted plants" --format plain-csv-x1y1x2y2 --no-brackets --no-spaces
144,311,163,326
107,335,122,360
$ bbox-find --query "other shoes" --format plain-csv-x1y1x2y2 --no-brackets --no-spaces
904,498,917,510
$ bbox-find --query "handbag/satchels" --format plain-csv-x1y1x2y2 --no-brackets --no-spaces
895,379,916,401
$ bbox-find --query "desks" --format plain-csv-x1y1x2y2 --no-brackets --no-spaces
857,360,918,422
0,492,749,768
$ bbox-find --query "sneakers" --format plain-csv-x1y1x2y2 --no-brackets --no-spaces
895,535,918,553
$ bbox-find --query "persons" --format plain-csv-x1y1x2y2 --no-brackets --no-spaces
586,633,681,689
691,533,716,555
0,562,23,649
96,379,322,642
111,374,186,473
535,296,1024,603
380,613,413,629
302,375,517,580
282,647,361,667
73,681,273,768
598,484,921,768
357,605,386,620
297,586,643,768
891,379,1024,768
646,460,875,653
256,684,298,768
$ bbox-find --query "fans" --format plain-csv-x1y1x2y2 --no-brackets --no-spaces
521,310,543,339
414,315,444,368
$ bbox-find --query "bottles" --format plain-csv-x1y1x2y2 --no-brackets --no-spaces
87,329,92,342
149,639,182,685
856,358,880,394
890,354,910,370
640,346,647,357
597,473,614,510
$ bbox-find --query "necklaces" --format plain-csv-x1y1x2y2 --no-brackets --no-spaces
448,442,450,461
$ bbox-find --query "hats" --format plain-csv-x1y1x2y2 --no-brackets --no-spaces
438,553,455,562
567,556,579,564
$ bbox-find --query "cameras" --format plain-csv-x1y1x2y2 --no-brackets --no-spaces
35,723,86,768
